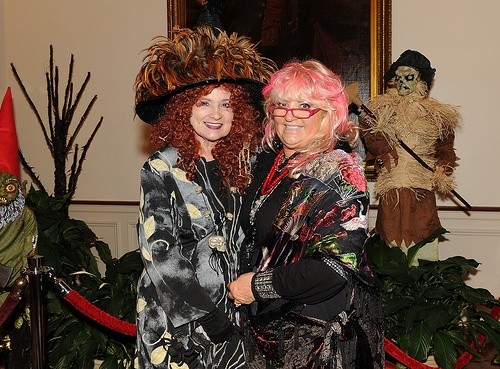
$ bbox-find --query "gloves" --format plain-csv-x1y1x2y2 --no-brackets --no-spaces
197,306,236,345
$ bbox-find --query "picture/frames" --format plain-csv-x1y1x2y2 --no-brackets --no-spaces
166,0,394,181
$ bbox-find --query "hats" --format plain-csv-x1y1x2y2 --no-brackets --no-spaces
133,25,280,126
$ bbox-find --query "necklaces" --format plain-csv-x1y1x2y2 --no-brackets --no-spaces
246,148,303,259
193,153,236,252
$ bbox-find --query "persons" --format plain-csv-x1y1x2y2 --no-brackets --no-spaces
228,60,384,369
133,28,279,369
357,49,462,267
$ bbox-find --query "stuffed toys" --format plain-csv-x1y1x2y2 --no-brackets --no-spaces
0,86,38,326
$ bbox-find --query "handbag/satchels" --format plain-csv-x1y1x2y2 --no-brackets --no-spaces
240,309,348,369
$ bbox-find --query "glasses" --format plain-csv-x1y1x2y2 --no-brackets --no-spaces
268,103,321,119
